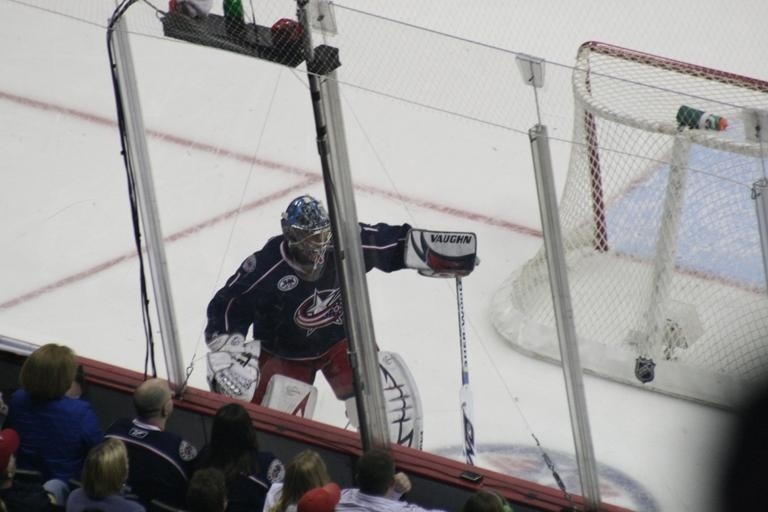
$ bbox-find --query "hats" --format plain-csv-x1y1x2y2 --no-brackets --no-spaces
0,428,20,471
297,482,341,512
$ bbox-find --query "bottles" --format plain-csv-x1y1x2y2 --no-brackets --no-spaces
675,105,728,132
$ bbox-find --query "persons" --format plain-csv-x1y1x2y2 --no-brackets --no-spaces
463,490,508,512
1,343,435,510
201,193,481,454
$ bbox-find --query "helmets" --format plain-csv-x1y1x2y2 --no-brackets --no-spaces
281,195,333,265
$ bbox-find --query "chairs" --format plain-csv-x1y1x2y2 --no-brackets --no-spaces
0,470,183,512
